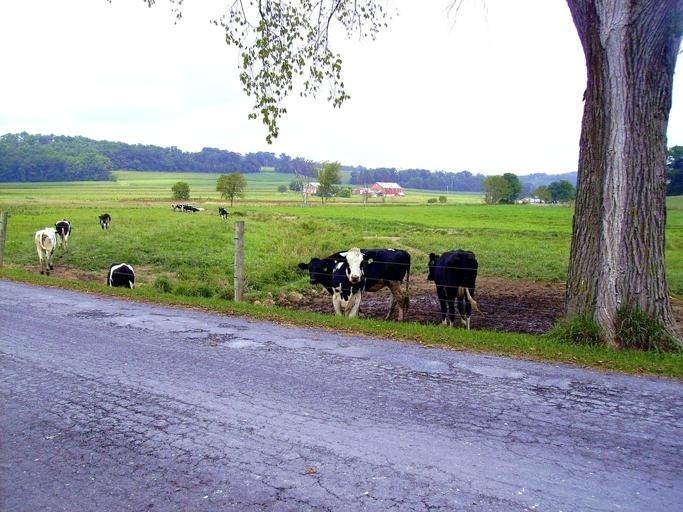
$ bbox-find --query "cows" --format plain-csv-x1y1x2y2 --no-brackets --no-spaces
33,227,60,277
425,247,480,331
55,219,72,249
296,247,413,321
218,207,229,220
98,213,111,229
106,260,137,290
172,202,200,214
